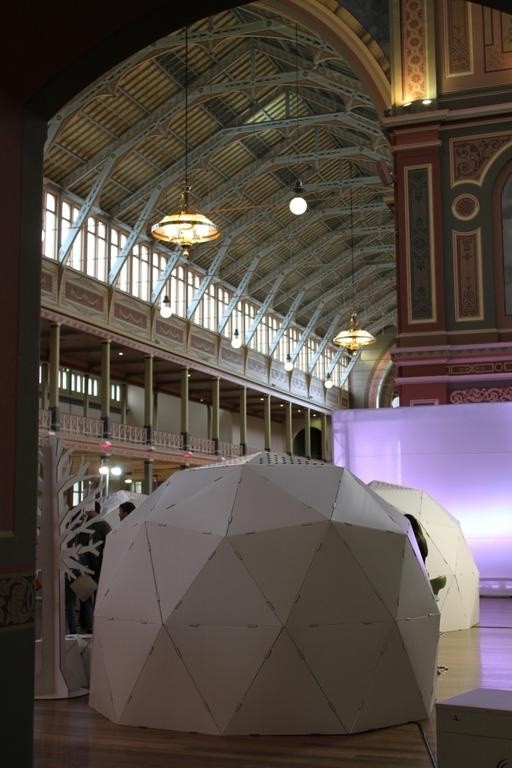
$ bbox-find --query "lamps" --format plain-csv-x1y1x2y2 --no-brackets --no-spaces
284,354,294,371
324,372,333,389
150,0,222,257
289,24,307,215
331,161,376,356
231,328,242,349
160,283,172,319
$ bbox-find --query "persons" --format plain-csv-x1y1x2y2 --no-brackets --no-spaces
65,500,136,633
